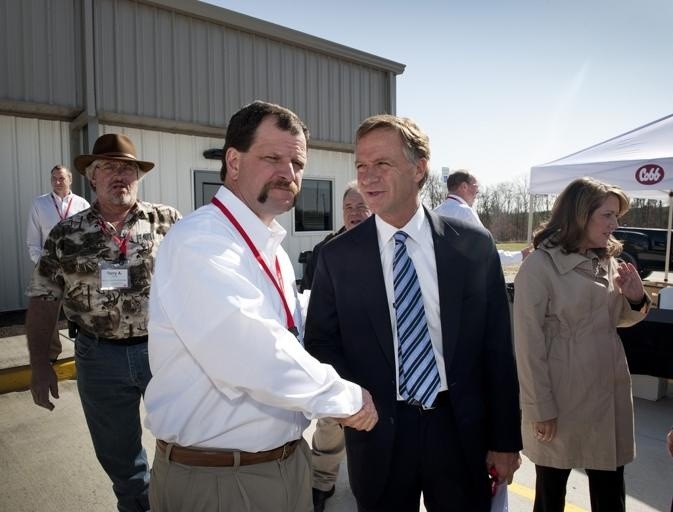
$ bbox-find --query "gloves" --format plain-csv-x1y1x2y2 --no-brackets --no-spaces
25,297,59,410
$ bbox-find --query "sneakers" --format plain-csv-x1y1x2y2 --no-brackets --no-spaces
312,485,336,512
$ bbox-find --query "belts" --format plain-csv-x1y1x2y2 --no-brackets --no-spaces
155,439,299,467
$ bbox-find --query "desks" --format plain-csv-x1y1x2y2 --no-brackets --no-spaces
503,282,672,401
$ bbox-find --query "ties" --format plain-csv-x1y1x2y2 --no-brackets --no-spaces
391,231,440,412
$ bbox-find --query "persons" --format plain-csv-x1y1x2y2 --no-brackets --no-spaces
28,134,182,512
304,115,524,512
432,171,496,239
296,181,372,511
515,178,652,512
145,101,378,512
25,166,91,338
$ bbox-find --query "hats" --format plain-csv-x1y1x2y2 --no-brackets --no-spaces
72,134,154,176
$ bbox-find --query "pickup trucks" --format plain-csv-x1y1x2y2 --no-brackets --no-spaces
612,226,672,279
